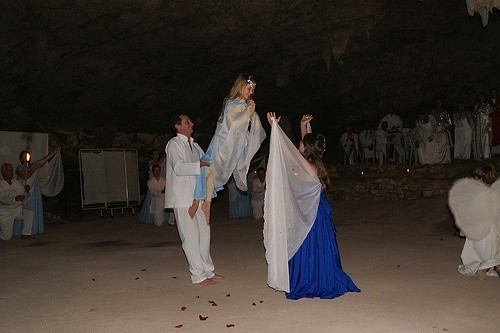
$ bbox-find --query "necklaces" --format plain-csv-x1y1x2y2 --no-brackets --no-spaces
0,163,35,241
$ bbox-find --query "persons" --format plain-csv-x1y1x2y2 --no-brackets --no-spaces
250,166,266,220
449,164,500,277
187,72,266,226
147,165,174,226
376,105,403,165
340,124,361,165
265,111,365,299
453,102,474,163
360,122,376,163
140,149,169,224
165,113,224,284
413,94,454,167
14,147,64,236
487,89,500,157
229,175,253,219
473,91,492,161
